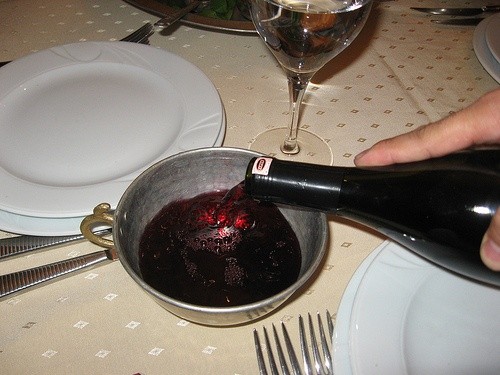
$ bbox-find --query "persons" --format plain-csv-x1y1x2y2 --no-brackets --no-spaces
354,88,500,271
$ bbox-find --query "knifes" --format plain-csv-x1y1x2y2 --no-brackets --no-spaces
410,5,500,15
431,18,484,25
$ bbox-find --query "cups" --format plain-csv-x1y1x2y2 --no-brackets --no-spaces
80,147,329,325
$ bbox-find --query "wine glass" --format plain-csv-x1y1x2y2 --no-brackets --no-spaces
247,0,373,166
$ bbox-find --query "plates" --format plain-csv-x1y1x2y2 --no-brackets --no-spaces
471,12,500,85
332,236,500,375
122,0,257,32
0,39,227,237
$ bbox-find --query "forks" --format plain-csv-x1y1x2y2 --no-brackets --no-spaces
252,309,334,375
116,22,154,46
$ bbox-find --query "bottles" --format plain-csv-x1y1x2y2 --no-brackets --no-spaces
244,141,500,289
